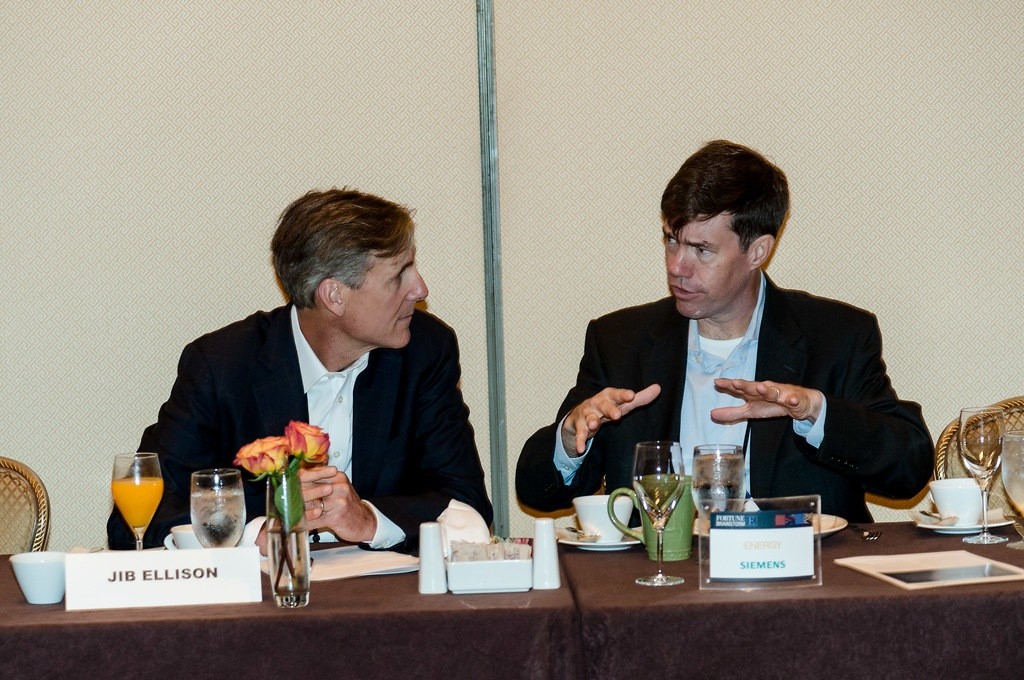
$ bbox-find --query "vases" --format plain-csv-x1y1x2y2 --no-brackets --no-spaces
265,472,310,609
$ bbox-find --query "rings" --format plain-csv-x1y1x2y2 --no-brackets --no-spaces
319,498,324,513
764,385,780,403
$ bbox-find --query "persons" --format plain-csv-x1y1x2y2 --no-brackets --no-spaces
515,139,937,524
107,188,494,557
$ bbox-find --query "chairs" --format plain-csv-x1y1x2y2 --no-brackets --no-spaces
0,396,1024,557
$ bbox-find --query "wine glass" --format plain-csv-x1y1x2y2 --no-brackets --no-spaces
957,407,1010,544
112,453,164,551
632,442,684,586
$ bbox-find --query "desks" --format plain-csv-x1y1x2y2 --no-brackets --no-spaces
562,519,1024,680
0,547,586,680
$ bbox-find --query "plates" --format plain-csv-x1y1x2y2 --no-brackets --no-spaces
164,533,177,550
554,516,641,550
631,514,848,539
917,520,1015,533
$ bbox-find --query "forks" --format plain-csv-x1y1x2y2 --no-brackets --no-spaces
847,524,883,541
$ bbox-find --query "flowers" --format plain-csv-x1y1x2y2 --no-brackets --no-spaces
234,419,329,601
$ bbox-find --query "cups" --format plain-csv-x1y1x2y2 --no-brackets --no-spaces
9,551,65,604
172,525,204,548
607,474,695,561
690,444,746,521
1002,430,1024,519
929,478,982,526
190,469,246,548
573,495,633,543
